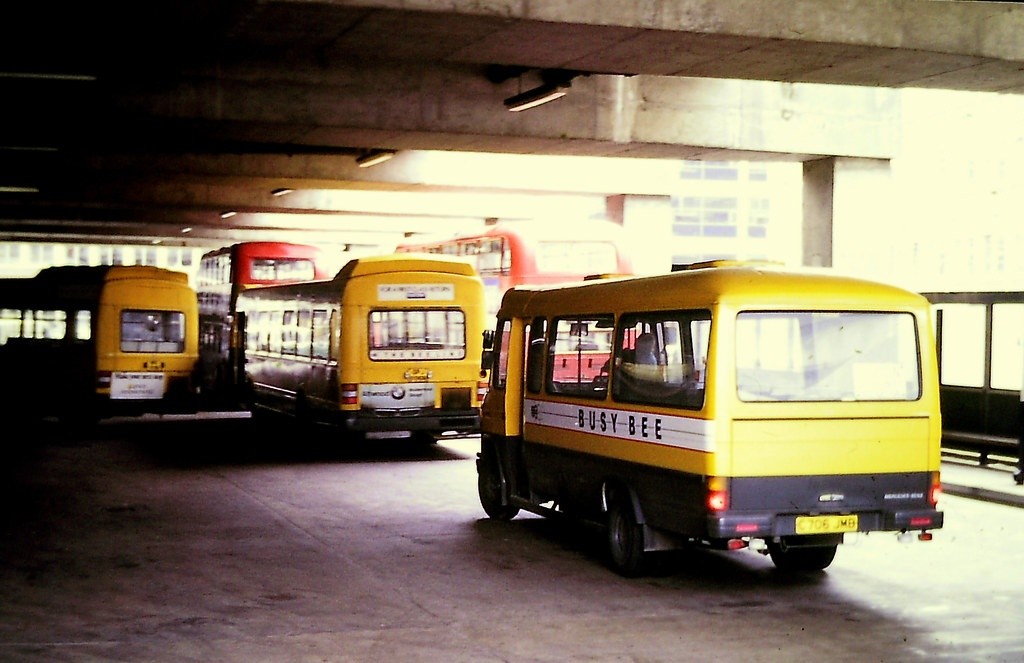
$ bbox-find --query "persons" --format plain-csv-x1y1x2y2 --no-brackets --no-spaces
188,352,227,390
635,332,658,366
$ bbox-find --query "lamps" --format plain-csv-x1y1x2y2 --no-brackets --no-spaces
355,149,399,168
220,211,238,219
180,226,192,234
502,69,569,112
271,188,296,196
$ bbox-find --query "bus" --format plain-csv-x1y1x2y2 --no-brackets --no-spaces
17,263,203,420
228,252,492,460
473,258,946,581
391,221,640,436
190,241,339,398
